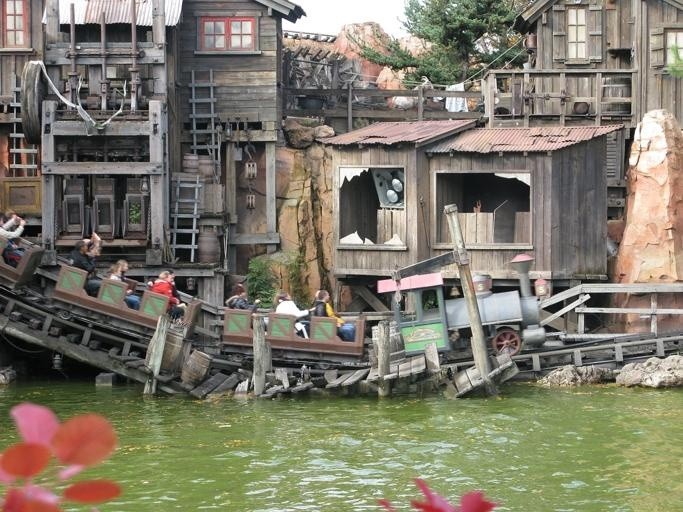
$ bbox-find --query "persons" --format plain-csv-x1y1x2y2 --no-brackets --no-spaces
1,211,189,324
223,281,355,345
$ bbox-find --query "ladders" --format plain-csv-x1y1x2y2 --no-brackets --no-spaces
168,175,202,263
188,68,220,173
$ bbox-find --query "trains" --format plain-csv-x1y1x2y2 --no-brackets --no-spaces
0,196,553,383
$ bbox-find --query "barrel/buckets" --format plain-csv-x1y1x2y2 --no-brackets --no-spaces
183,154,199,175
601,77,631,114
370,321,407,369
179,349,213,386
198,232,220,263
144,329,184,375
574,102,589,115
200,155,214,177
